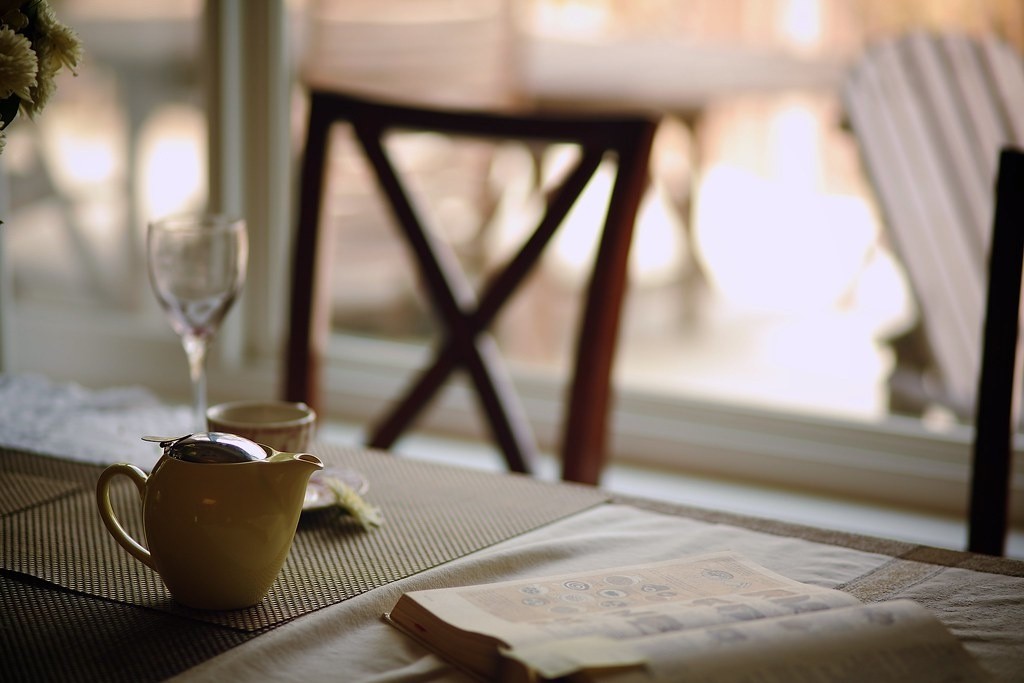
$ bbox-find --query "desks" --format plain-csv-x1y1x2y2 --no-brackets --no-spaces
0,366,1024,683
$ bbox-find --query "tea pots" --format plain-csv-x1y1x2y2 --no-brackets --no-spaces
97,431,324,611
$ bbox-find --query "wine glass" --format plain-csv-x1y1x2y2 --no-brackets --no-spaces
147,214,246,428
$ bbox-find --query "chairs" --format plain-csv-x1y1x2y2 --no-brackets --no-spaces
966,146,1024,558
282,77,659,490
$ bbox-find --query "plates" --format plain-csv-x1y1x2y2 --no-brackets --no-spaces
303,467,367,512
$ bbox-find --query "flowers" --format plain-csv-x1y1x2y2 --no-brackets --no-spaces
325,474,386,534
0,0,84,155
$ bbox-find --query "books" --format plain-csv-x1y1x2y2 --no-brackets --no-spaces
383,551,991,683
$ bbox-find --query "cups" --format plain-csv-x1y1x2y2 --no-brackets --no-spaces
203,402,314,453
